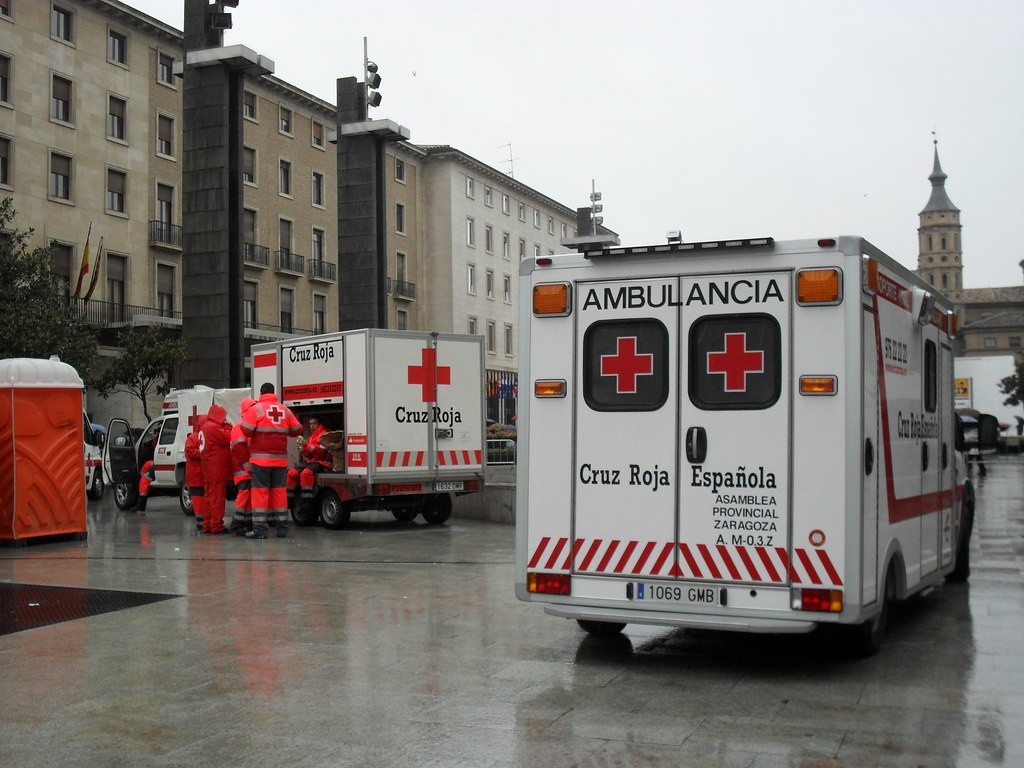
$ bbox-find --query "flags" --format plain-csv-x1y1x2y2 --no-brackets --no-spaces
71,236,106,303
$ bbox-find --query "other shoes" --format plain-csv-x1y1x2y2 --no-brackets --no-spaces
212,528,230,534
277,529,286,537
296,500,314,515
244,530,267,539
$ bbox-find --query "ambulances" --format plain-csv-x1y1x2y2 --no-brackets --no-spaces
516,235,999,659
83,409,104,501
250,328,487,529
101,387,251,517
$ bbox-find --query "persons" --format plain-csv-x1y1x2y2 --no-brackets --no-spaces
968,440,986,477
185,382,333,539
132,427,159,515
